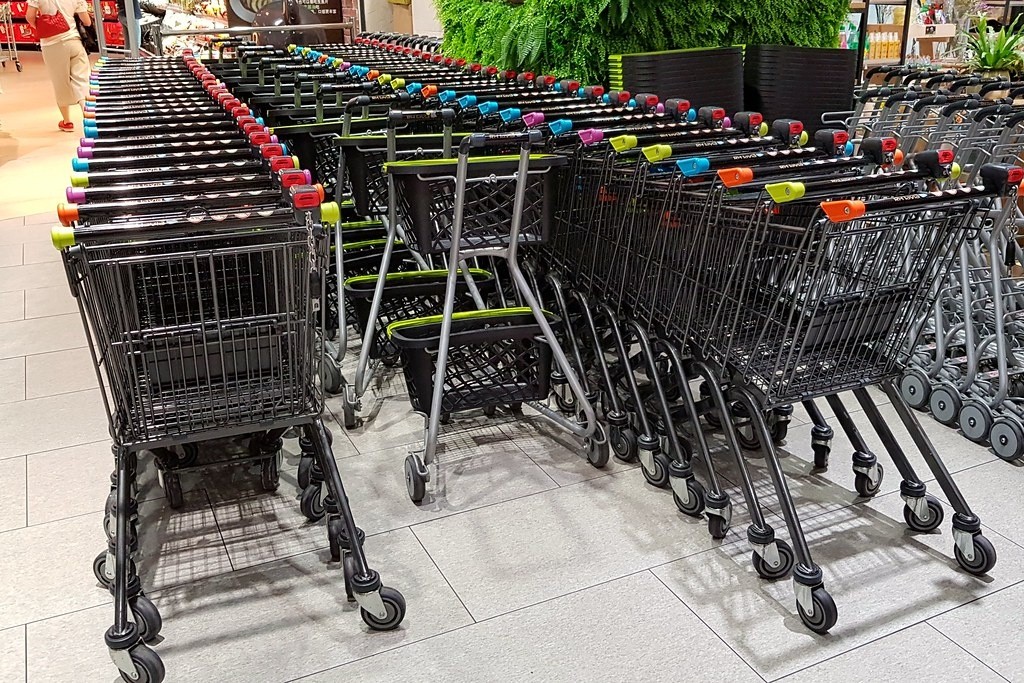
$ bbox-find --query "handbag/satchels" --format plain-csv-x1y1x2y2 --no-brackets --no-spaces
35,0,71,39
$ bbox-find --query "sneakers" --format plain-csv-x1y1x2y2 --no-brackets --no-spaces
58,121,74,131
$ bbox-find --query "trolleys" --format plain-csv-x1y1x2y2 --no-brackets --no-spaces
0,1,24,72
50,31,607,683
286,31,1024,633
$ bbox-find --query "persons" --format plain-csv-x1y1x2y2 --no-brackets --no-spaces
26,0,91,131
119,0,142,58
